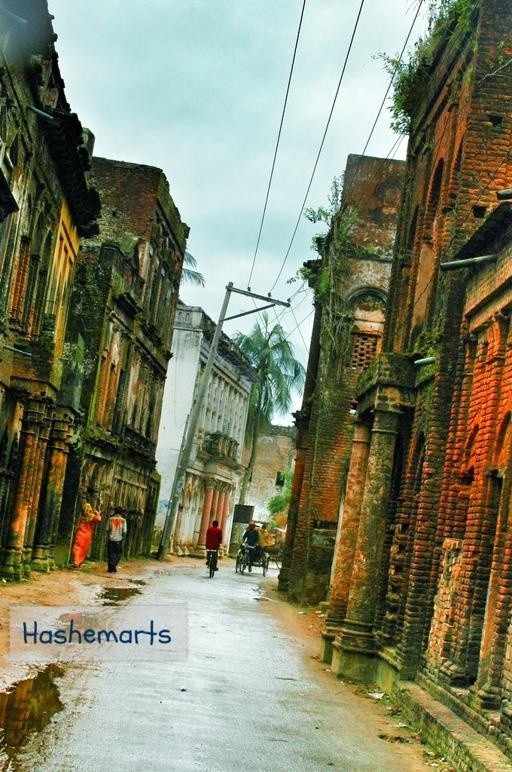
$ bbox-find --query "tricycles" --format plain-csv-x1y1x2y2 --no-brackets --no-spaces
234,543,272,577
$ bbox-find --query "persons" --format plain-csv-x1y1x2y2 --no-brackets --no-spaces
105,506,129,573
72,502,102,566
242,521,260,574
204,519,223,571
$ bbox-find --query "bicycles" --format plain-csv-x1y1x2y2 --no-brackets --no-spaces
205,549,216,579
